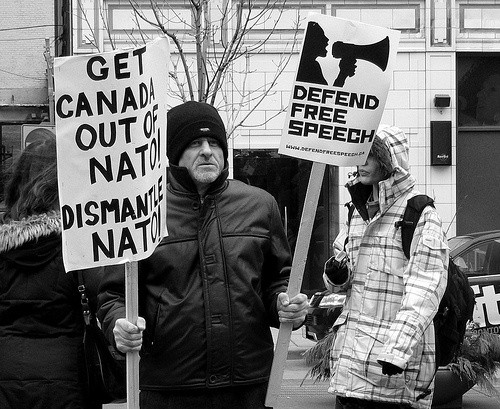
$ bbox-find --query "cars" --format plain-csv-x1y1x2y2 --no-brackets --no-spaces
302,229,500,345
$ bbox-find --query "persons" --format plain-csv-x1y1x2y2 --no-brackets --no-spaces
1,128,106,409
96,99,312,408
322,124,450,409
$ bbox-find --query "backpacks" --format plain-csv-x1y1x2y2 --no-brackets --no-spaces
345,195,476,367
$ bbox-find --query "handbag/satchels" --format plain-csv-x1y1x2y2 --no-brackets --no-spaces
82,322,126,404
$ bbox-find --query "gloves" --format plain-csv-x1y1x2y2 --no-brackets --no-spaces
325,257,348,285
382,362,404,376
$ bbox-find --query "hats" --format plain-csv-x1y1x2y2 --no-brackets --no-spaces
167,101,229,166
